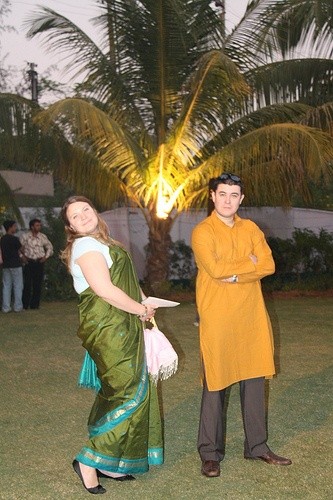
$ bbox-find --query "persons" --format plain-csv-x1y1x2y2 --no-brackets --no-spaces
192,173,291,478
0,219,53,313
58,195,157,494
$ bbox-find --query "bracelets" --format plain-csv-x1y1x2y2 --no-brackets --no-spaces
136,305,148,317
232,274,237,283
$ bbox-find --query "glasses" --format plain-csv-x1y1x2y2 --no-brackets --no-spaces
217,173,241,183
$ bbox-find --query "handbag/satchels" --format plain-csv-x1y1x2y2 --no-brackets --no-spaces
142,318,179,383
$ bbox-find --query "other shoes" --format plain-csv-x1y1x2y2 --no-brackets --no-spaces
201,460,220,476
4,308,26,313
244,449,292,465
72,458,106,495
96,469,136,481
24,305,39,310
